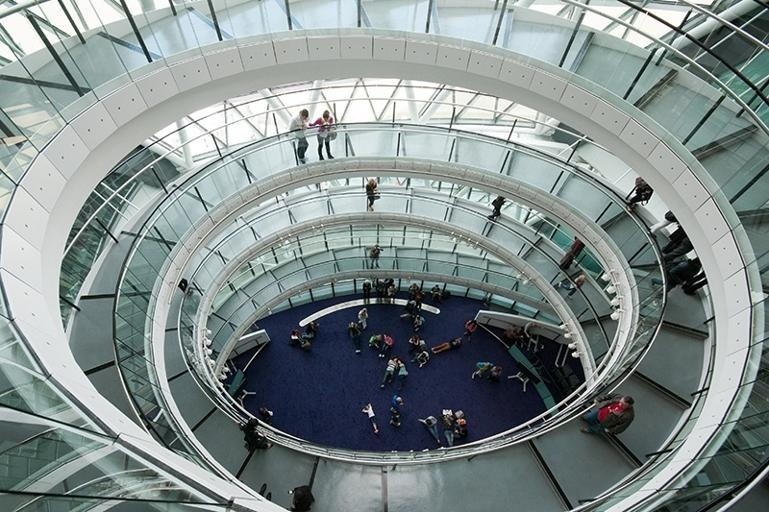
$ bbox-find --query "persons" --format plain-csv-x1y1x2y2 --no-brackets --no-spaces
289,244,527,446
286,484,315,512
178,278,193,296
581,393,634,436
308,110,335,160
647,210,708,298
487,195,506,220
365,179,377,211
627,184,654,212
239,406,274,451
559,275,585,300
288,110,310,165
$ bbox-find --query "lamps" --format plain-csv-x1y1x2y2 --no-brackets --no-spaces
516,270,531,285
602,268,627,320
215,363,230,389
560,324,583,359
203,329,216,369
448,231,479,252
